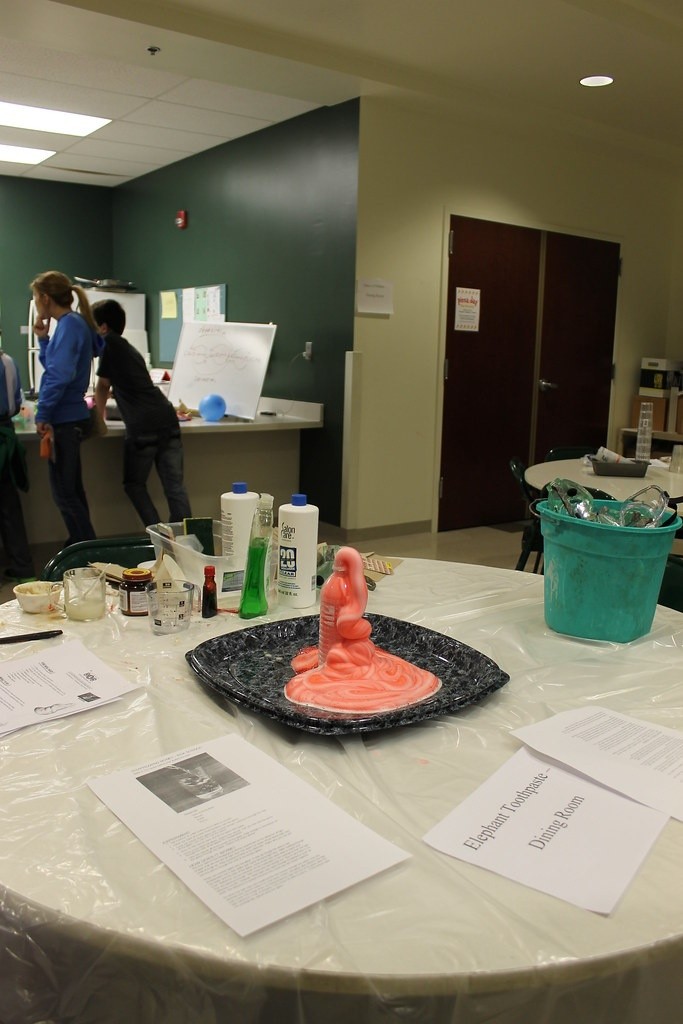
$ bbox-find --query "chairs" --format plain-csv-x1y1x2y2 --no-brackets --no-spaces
509,456,544,575
40,535,157,583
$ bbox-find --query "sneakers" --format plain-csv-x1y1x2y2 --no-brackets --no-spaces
4,566,38,583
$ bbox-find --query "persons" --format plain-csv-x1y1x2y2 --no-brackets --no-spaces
91,298,192,527
0,350,37,583
31,271,106,548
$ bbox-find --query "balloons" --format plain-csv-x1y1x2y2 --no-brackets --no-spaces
199,394,226,422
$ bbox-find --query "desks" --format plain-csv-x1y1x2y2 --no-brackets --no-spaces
0,556,682,1023
525,458,683,504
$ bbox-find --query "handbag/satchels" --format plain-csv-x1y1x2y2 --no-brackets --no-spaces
77,405,107,443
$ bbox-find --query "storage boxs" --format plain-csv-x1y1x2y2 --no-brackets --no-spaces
639,357,683,397
632,394,669,433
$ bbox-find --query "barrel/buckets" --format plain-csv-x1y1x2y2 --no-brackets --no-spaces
528,496,683,643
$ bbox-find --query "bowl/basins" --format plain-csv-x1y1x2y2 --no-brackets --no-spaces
13,581,61,613
587,456,652,477
145,521,269,600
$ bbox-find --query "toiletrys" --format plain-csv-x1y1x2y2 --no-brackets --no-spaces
237,492,274,620
219,481,260,620
276,493,321,609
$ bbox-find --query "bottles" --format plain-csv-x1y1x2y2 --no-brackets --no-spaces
119,567,157,616
276,493,317,609
201,566,218,619
239,491,275,621
219,481,261,556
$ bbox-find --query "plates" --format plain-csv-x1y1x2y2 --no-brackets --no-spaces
184,612,511,735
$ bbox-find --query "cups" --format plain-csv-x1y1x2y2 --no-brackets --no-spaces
144,579,195,636
63,566,105,621
669,445,683,474
635,402,654,460
21,402,34,430
